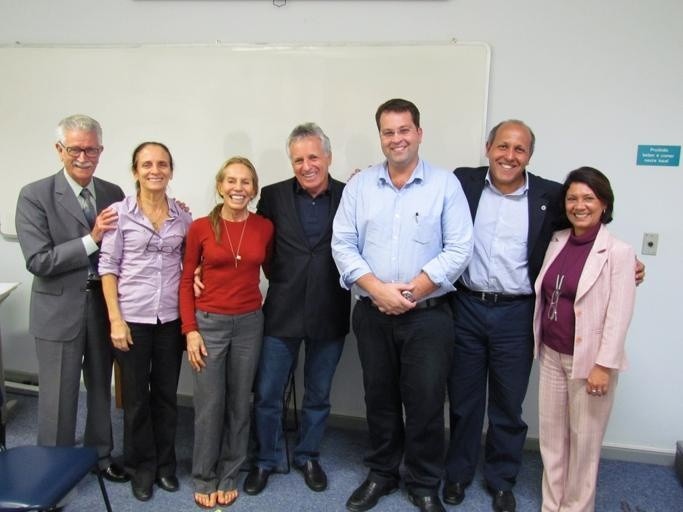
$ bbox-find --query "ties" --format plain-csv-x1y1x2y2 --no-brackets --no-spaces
80,189,102,277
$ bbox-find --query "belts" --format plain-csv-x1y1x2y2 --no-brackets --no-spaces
360,295,437,309
86,280,101,292
454,280,532,302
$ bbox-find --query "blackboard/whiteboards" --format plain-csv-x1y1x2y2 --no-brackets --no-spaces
0,41,491,240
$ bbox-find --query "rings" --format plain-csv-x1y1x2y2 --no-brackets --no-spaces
597,390,601,393
591,389,596,393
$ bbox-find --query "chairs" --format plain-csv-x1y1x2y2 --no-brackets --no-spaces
0,420,113,512
279,342,302,475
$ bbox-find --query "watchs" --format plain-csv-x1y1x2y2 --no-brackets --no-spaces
401,290,416,303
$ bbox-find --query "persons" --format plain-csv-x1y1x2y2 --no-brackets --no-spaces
99,142,194,499
348,119,645,511
330,100,475,511
178,158,274,510
532,165,636,511
193,122,353,495
14,114,133,512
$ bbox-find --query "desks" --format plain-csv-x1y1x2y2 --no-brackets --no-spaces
1,281,23,432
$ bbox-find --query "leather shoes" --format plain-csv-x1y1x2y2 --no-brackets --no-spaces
243,464,271,495
345,467,400,512
407,486,446,512
91,459,179,502
481,478,516,511
292,459,326,491
443,479,473,505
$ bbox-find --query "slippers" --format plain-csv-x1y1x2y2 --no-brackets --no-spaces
193,491,238,509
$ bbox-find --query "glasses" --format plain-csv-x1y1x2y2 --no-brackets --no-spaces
59,141,100,158
547,289,561,322
145,223,180,253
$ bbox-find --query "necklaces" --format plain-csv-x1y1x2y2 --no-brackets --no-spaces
221,213,247,269
152,217,161,229
